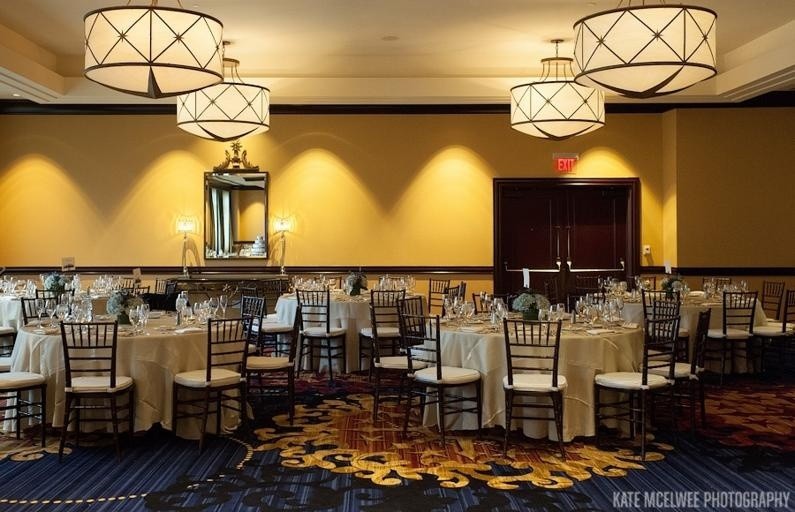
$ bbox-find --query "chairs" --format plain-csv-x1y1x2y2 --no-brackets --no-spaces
0,275,795,462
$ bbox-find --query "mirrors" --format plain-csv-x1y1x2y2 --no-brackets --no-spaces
203,139,269,260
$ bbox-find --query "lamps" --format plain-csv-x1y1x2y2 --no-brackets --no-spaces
82,0,225,99
176,57,271,142
572,0,718,99
509,39,606,140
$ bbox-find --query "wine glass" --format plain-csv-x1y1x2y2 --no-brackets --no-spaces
620,275,751,303
176,290,227,327
442,291,510,330
0,274,48,302
290,273,353,302
538,302,565,335
128,303,149,336
69,272,123,298
570,292,624,332
371,272,417,300
34,298,93,336
